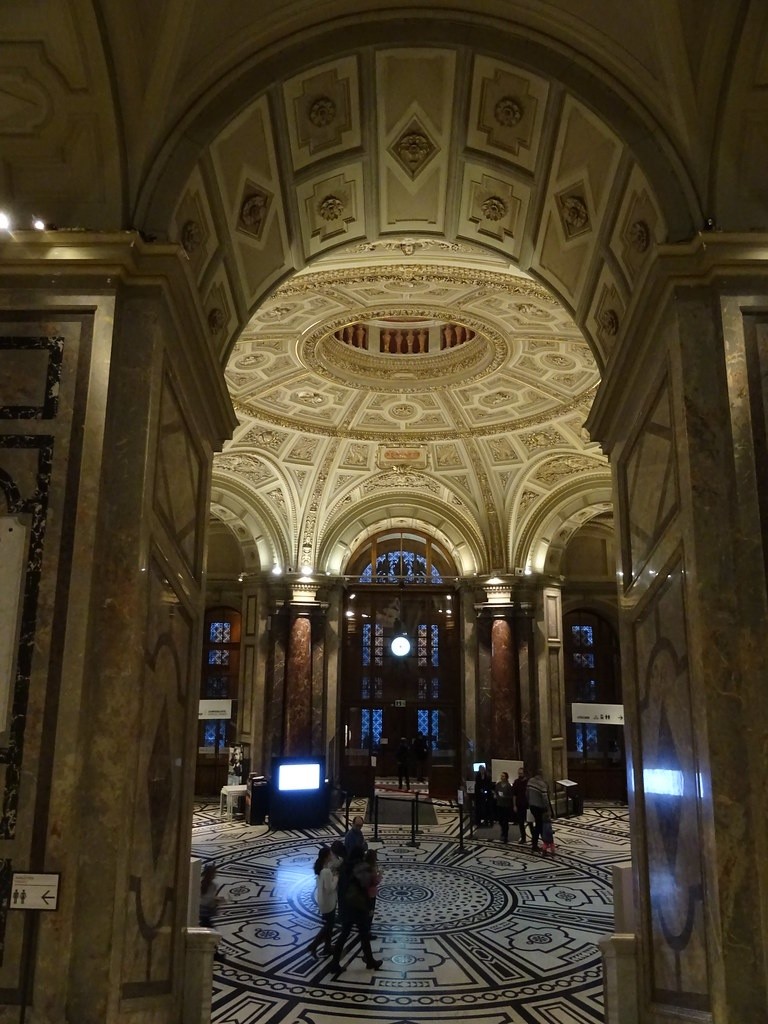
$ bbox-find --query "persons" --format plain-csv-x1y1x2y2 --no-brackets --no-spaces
475,766,560,858
199,863,226,961
307,816,383,974
396,738,411,789
413,731,428,782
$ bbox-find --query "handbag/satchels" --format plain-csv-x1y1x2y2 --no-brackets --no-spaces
526,809,536,822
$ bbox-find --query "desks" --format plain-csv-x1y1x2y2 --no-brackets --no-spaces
219,784,247,822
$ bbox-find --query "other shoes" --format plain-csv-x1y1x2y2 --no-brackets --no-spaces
369,935,377,941
324,945,334,952
307,945,319,959
532,847,540,852
331,965,346,974
366,960,383,969
519,839,526,844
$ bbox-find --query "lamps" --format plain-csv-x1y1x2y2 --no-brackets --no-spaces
391,579,411,657
